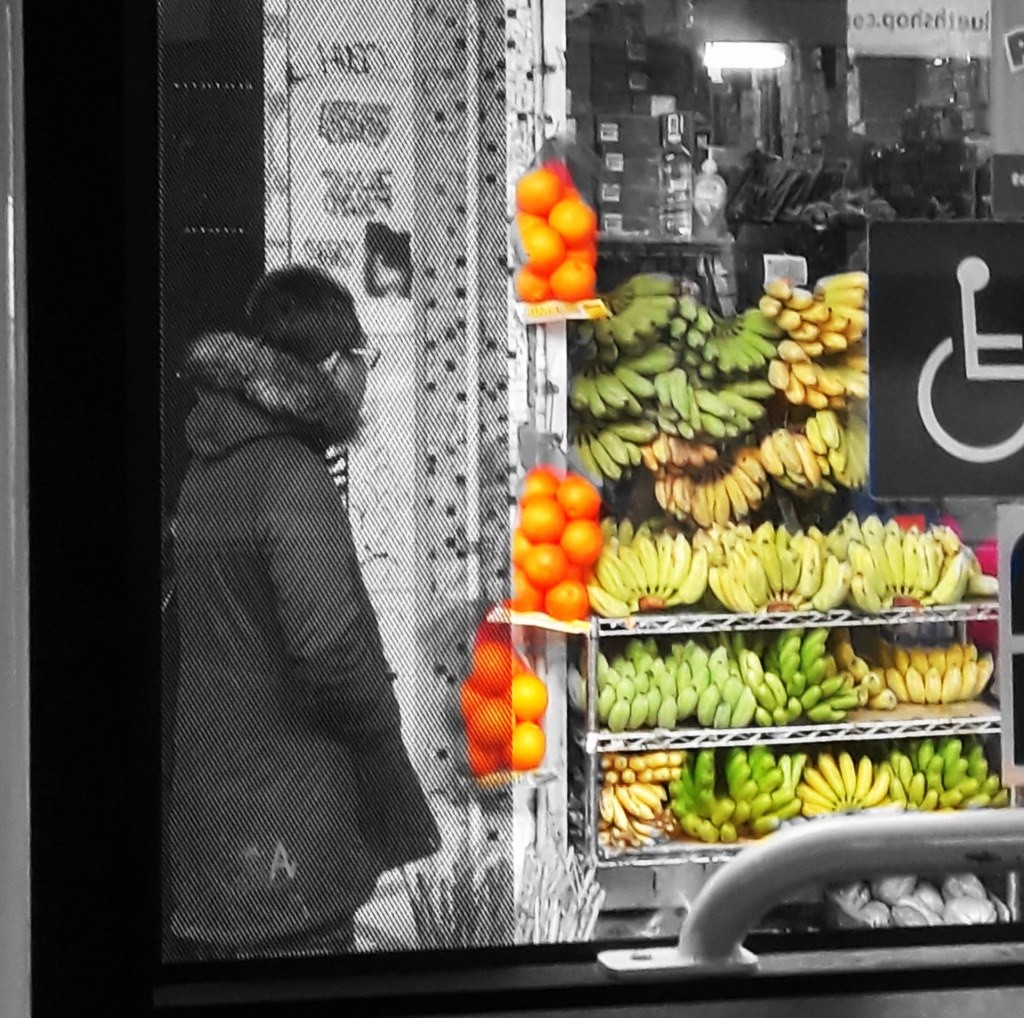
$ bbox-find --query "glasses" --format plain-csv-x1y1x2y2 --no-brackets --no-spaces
342,346,383,370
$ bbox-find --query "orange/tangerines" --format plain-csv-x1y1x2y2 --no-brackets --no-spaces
510,163,597,305
506,465,605,623
447,642,548,778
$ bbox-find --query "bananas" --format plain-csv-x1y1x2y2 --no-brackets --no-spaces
570,269,1014,854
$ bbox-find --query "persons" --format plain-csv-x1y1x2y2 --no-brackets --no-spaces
173,264,442,955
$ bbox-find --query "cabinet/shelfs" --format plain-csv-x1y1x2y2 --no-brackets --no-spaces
486,584,1017,880
